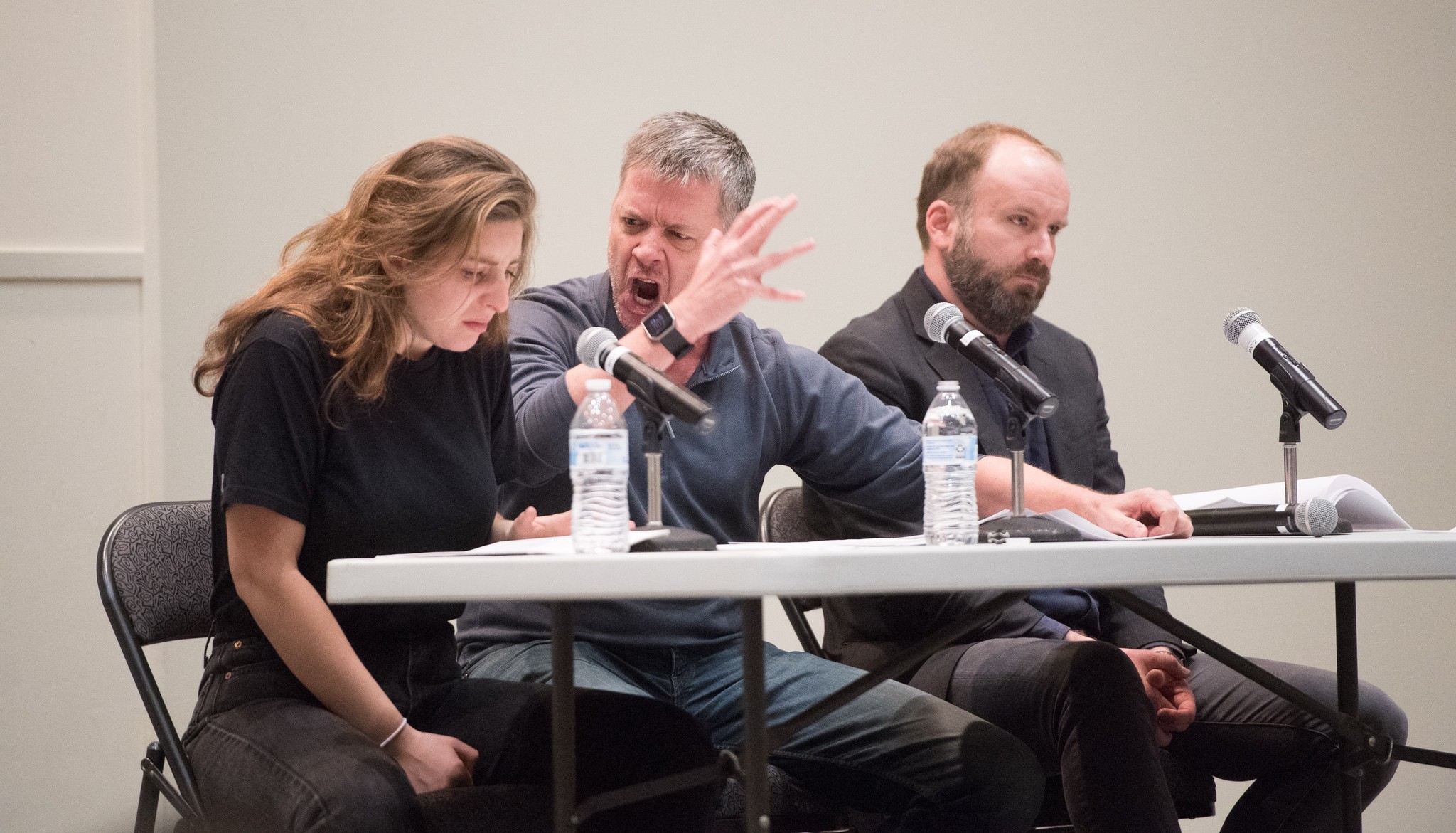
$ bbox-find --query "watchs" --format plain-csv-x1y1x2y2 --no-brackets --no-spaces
640,302,695,361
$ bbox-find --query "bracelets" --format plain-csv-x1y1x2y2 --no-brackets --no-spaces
380,717,407,747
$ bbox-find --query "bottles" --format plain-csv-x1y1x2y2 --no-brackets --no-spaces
566,376,634,555
921,380,980,547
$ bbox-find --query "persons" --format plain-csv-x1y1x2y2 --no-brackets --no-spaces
806,124,1408,833
457,111,1194,833
182,135,714,833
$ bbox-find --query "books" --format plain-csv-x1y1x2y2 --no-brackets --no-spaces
1160,474,1413,530
378,530,670,554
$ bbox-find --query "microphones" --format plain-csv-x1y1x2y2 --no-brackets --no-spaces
1180,497,1338,538
575,326,718,434
1222,307,1346,429
924,302,1060,418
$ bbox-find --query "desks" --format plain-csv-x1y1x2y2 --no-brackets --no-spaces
327,526,1456,833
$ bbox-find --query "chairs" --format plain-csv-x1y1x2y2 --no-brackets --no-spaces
97,502,216,833
